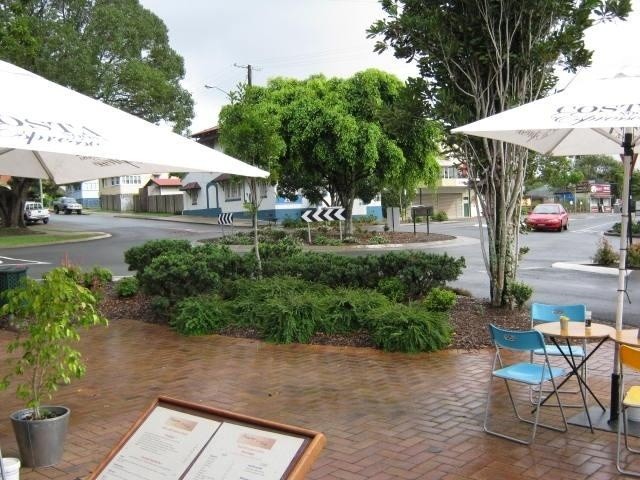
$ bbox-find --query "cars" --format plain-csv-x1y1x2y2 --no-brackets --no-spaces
525,203,569,231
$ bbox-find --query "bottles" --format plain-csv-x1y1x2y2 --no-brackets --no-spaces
584,311,591,327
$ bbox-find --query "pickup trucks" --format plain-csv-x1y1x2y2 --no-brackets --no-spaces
24,200,50,224
52,196,82,215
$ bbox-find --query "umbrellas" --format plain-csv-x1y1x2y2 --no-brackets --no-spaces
0,60,271,186
450,77,640,422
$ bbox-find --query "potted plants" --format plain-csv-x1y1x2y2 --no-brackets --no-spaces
1,265,111,467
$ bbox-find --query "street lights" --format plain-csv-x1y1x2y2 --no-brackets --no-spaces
204,84,235,103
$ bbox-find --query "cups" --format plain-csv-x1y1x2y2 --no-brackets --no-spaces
585,328,592,335
561,318,569,329
561,329,568,336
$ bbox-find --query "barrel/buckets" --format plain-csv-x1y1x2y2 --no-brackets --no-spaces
11,406,71,466
1,457,21,480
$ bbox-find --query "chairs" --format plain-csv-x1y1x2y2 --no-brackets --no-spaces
481,299,640,478
